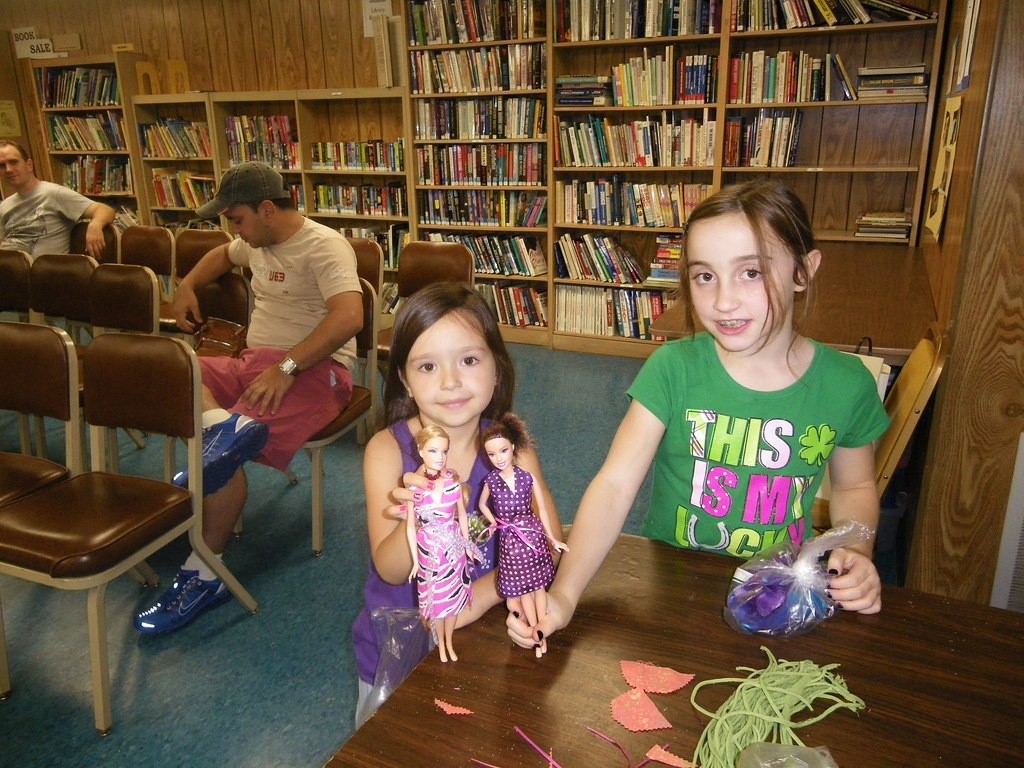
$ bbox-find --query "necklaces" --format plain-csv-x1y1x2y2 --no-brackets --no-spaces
424,469,441,481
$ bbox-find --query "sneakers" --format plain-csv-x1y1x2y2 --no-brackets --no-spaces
173,413,270,497
133,561,234,634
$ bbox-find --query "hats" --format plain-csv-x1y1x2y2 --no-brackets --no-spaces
195,161,292,220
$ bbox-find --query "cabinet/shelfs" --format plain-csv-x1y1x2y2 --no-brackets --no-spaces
15,51,151,235
400,0,549,346
131,86,414,330
550,0,948,359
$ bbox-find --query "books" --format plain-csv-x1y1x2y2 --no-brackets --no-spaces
404,0,939,342
33,66,410,316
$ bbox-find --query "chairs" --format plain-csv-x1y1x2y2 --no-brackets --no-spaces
0,221,476,737
809,320,949,541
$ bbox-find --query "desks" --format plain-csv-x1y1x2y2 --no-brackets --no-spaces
321,525,1023,767
648,243,941,366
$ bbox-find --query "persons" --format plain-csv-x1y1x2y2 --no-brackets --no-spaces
478,411,570,658
0,140,115,259
352,282,564,732
406,424,481,662
130,160,364,633
506,178,890,649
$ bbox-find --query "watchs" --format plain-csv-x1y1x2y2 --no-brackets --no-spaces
279,357,299,376
